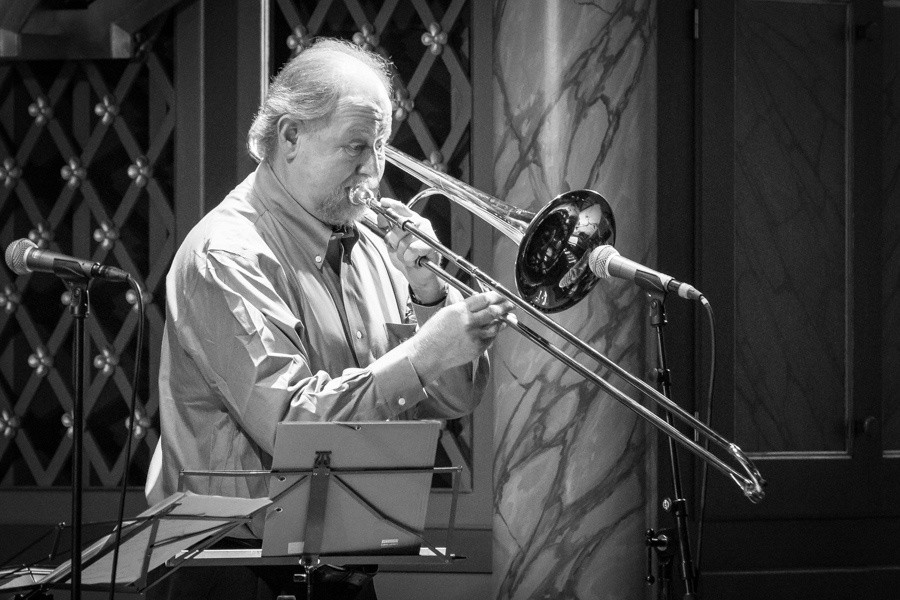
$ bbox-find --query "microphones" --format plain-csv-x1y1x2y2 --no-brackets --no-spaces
4,236,129,283
587,243,704,301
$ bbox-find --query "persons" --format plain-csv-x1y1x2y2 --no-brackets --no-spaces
145,36,519,600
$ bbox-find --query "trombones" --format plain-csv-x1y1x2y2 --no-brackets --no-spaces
348,142,771,505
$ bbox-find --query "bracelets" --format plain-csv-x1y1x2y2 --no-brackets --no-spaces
408,281,449,307
